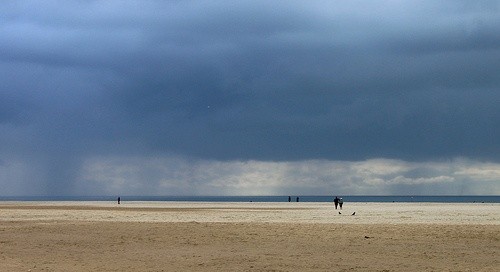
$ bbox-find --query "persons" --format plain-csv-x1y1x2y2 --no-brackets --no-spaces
118,196,120,204
334,197,340,209
289,196,291,202
339,198,343,209
297,197,299,202
